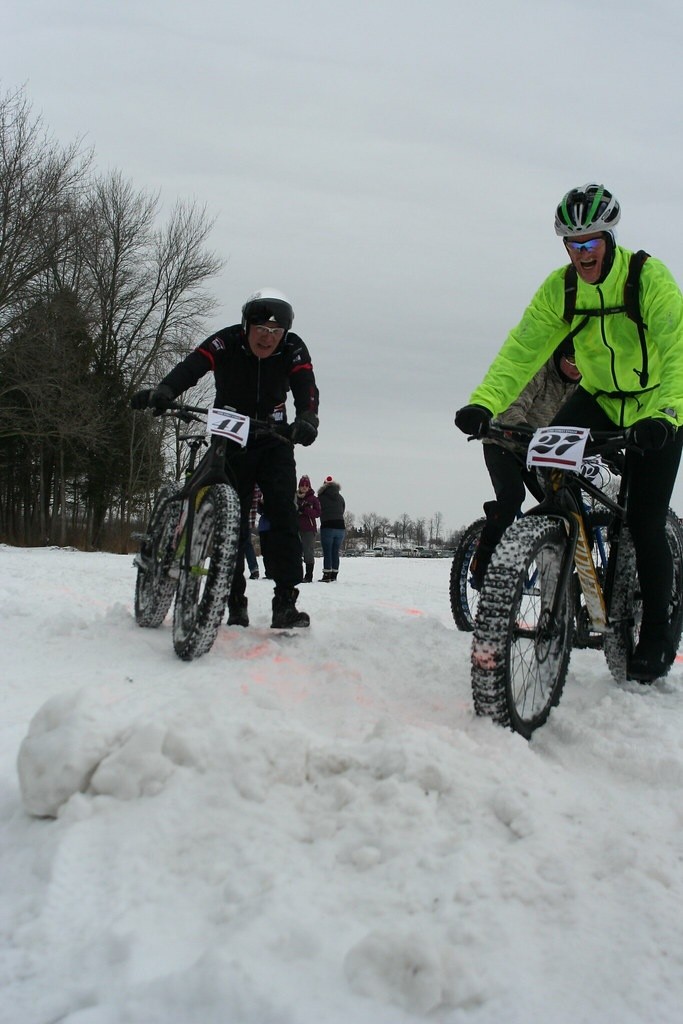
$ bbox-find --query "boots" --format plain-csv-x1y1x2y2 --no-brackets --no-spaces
301,563,314,583
319,569,331,582
330,570,339,580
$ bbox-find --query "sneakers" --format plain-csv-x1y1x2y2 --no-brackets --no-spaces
629,638,677,678
227,596,249,627
272,598,309,628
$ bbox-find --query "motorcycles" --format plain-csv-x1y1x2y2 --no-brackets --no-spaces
132,394,298,662
450,418,683,740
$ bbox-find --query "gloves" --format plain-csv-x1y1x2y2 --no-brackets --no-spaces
454,403,493,439
130,389,168,417
630,418,669,452
287,417,318,447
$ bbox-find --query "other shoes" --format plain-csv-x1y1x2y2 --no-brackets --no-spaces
249,571,259,579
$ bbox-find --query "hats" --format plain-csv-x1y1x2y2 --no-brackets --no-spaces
323,475,334,485
298,474,311,488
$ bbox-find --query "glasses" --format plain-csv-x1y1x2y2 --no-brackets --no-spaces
561,353,575,366
248,324,285,338
564,235,610,255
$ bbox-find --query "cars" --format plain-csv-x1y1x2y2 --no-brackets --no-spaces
339,546,450,558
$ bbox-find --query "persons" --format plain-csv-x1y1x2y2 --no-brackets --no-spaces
244,475,346,583
454,183,682,682
129,298,320,629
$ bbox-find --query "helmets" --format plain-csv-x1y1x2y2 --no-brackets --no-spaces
240,287,294,343
553,336,582,386
554,185,622,238
